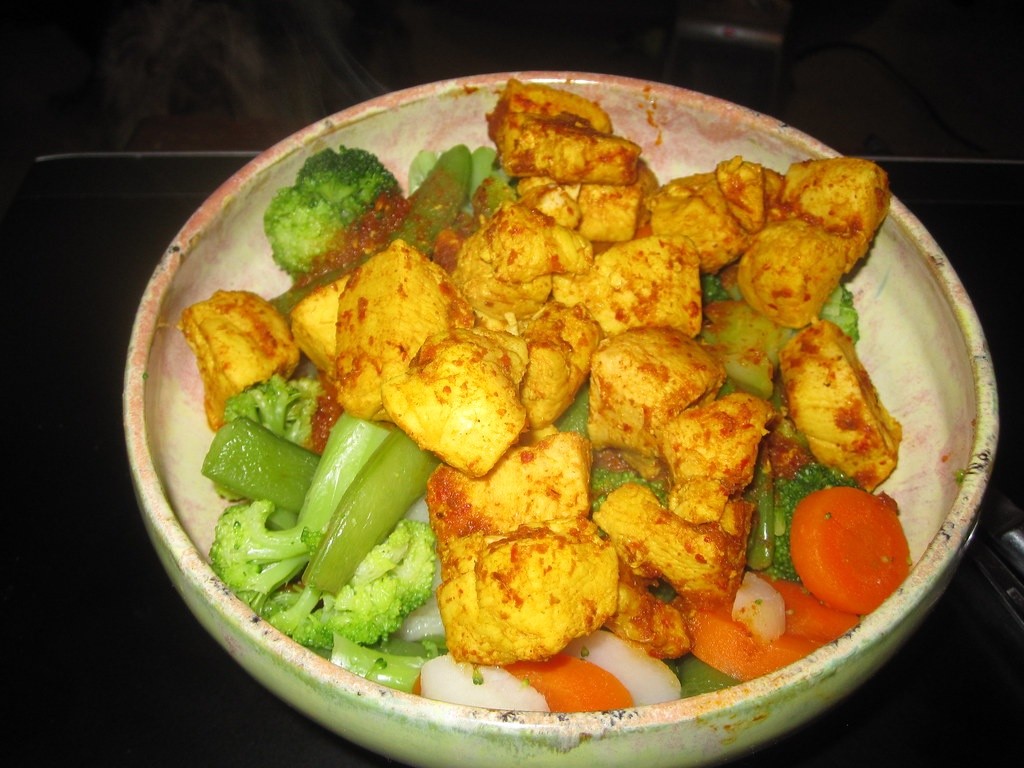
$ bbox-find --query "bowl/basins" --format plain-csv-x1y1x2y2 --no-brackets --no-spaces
125,71,999,768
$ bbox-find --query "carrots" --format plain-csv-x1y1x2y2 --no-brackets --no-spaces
795,488,939,593
502,657,632,724
676,574,864,683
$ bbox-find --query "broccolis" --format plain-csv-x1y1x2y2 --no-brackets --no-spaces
197,371,455,695
261,150,409,280
544,270,866,585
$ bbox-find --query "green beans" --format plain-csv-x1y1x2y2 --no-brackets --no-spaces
268,142,470,331
